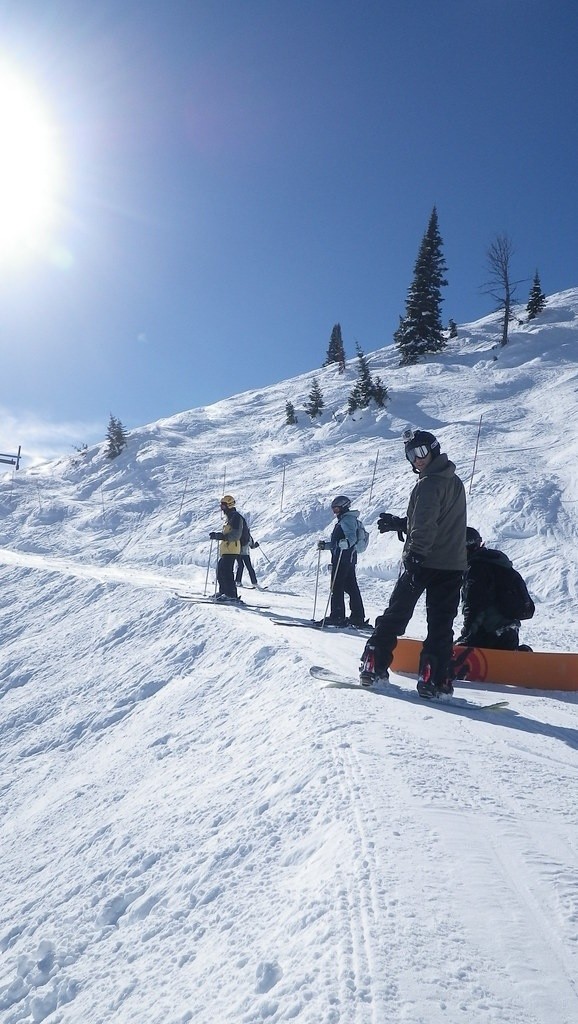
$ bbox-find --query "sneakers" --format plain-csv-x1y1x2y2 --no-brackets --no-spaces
417,654,454,700
322,617,346,628
348,618,363,628
359,643,393,686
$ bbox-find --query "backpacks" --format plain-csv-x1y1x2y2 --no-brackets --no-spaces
353,520,369,554
468,564,535,620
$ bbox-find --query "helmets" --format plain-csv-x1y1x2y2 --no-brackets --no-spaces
331,496,351,508
466,527,482,546
402,429,441,455
221,496,235,509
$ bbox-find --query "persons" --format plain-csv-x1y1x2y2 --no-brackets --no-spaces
209,495,243,601
358,430,468,699
234,536,260,586
319,496,365,627
454,527,533,652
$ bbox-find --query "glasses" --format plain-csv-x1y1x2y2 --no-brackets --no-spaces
406,445,429,463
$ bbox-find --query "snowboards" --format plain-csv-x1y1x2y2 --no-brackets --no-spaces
390,639,578,692
310,666,509,710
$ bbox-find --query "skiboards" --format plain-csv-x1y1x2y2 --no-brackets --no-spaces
171,592,271,609
212,581,268,591
270,617,375,635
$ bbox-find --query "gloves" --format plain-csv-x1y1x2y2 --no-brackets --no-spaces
210,532,215,539
339,539,349,549
318,540,325,549
255,542,259,548
377,513,407,542
215,533,224,540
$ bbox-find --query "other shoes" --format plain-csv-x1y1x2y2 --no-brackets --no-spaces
209,592,222,598
213,594,237,604
518,644,532,651
